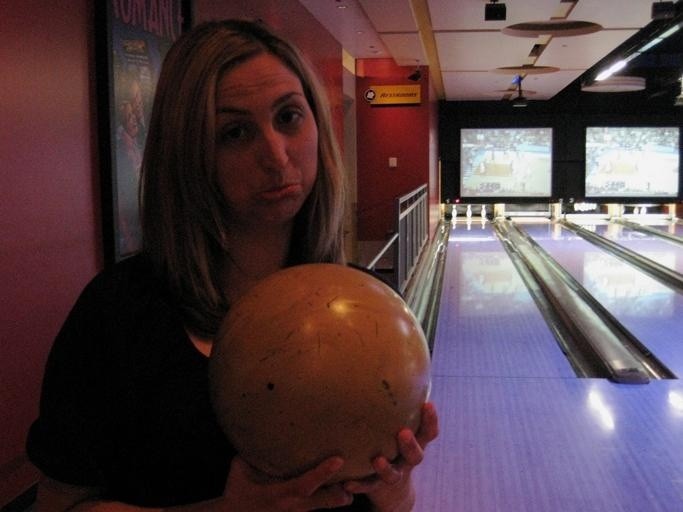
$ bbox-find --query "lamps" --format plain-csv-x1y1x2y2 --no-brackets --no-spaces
511,77,527,108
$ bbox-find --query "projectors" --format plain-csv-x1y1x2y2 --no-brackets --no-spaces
675,95,683,106
513,98,527,107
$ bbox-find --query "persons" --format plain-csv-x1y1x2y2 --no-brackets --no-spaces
117,101,152,254
120,68,150,140
23,12,440,512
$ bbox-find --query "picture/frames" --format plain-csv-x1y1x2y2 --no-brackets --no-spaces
101,0,196,266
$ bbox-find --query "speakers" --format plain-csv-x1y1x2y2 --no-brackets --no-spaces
485,4,506,20
652,2,674,19
